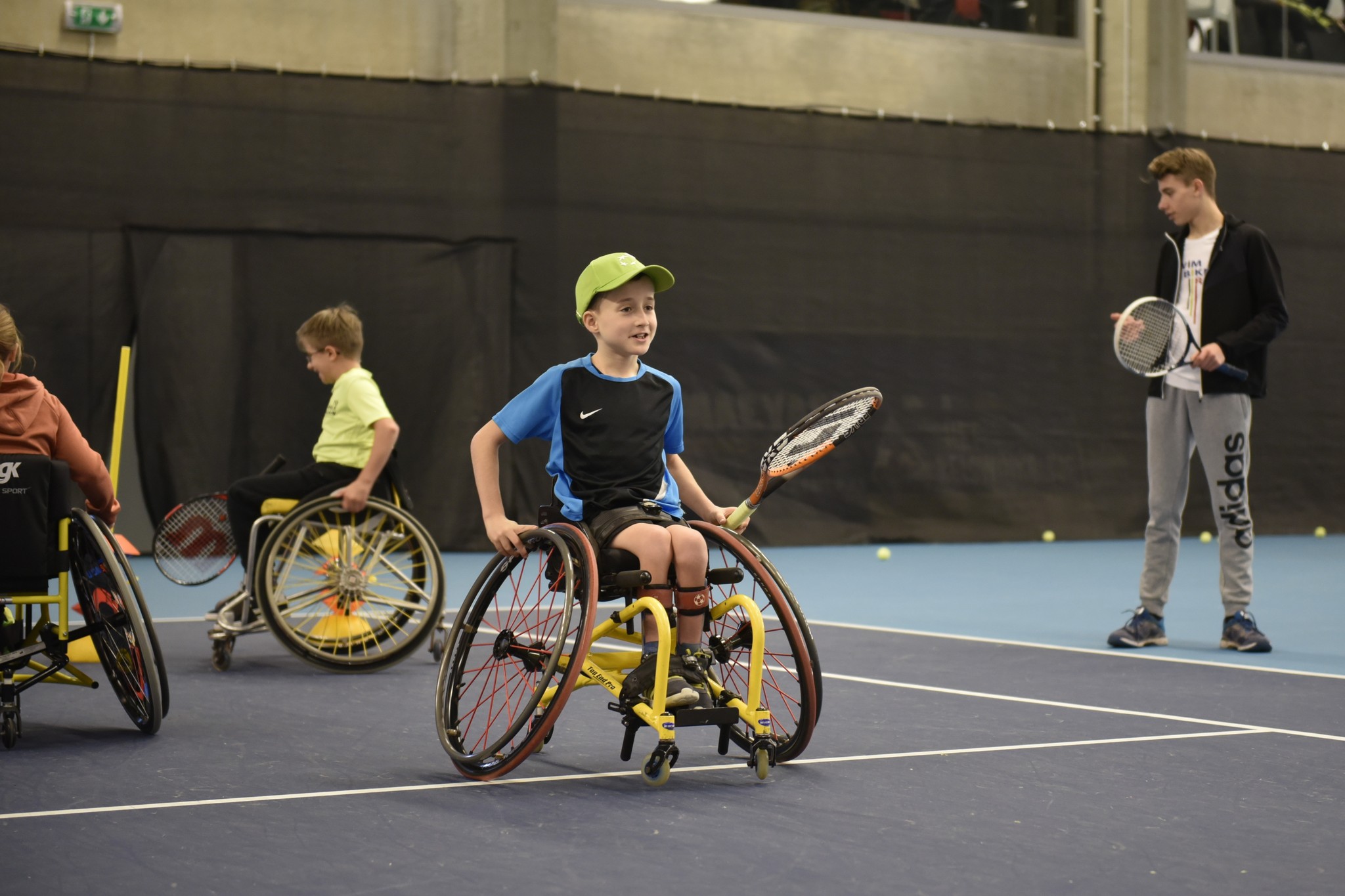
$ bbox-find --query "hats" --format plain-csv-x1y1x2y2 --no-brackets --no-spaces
575,251,675,327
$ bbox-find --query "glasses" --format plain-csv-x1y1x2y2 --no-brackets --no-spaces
304,348,341,362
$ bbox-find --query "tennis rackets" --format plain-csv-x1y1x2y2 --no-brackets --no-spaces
152,448,287,586
1113,297,1260,388
728,384,883,532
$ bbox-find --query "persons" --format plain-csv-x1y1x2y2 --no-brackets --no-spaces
207,305,400,642
1107,148,1287,651
0,305,121,535
469,253,751,709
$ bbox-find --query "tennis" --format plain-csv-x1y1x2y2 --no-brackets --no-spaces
1202,530,1212,544
1316,526,1326,539
1043,530,1056,542
876,547,890,559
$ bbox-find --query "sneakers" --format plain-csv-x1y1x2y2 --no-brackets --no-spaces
675,642,715,710
205,579,290,631
1107,605,1169,649
641,651,699,708
1219,610,1273,652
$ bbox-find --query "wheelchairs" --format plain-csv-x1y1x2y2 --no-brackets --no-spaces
0,455,169,737
435,524,823,780
208,496,450,672
